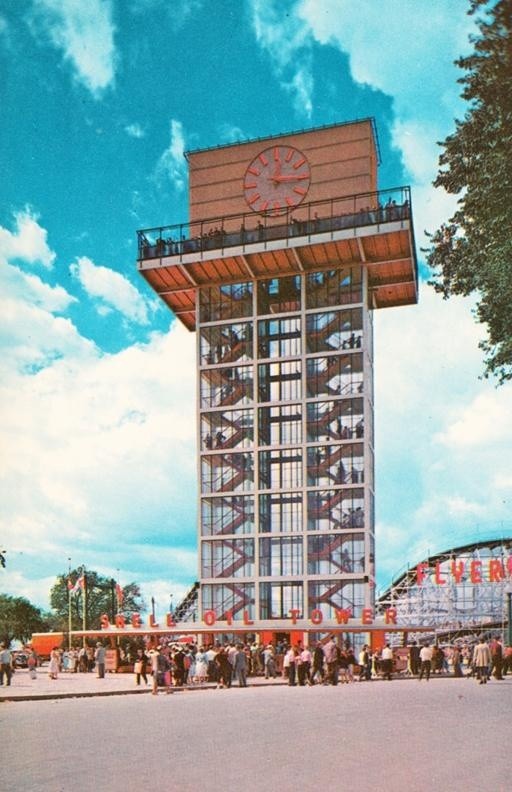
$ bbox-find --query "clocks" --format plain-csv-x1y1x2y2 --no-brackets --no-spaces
236,144,314,220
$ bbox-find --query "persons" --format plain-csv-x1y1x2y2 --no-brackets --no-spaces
139,198,411,572
0,632,511,693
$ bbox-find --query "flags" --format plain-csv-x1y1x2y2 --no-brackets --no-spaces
115,582,123,601
67,572,85,593
